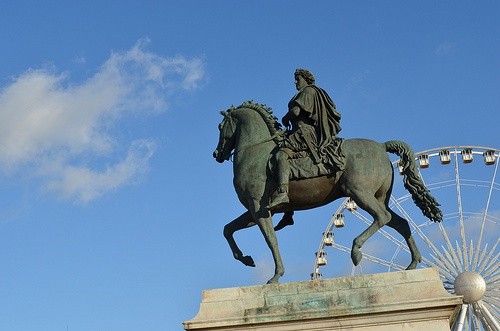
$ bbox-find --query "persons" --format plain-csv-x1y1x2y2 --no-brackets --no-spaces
264,68,342,232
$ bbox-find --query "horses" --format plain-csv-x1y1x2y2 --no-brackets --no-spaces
213,100,443,285
